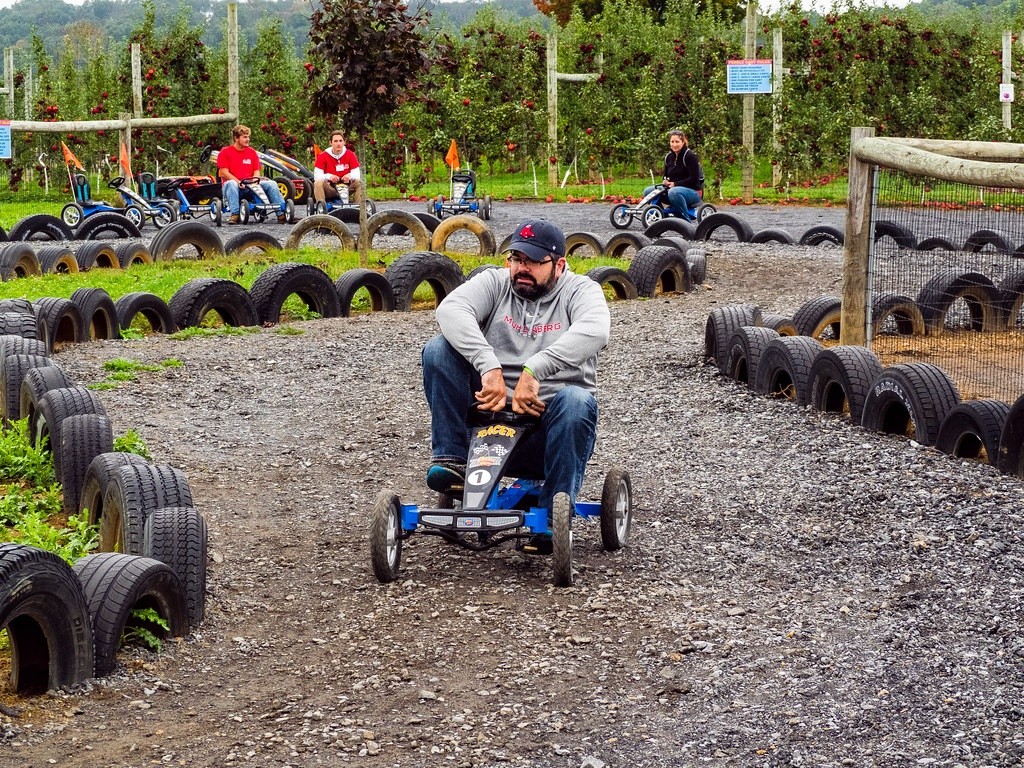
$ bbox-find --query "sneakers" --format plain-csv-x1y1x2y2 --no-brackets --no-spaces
426,462,465,501
529,532,554,554
278,215,301,223
228,214,240,224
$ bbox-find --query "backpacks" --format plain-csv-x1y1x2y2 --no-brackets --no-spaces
665,149,704,188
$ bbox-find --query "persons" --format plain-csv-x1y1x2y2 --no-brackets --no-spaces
642,130,702,223
217,126,302,224
422,218,612,555
313,131,367,208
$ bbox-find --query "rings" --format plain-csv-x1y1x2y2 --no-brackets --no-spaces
525,402,533,407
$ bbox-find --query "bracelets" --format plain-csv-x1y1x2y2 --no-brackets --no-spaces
523,366,535,378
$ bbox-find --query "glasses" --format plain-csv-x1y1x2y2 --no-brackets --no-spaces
507,252,559,269
670,131,683,138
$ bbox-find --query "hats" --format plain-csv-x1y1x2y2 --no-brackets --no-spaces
502,219,567,262
